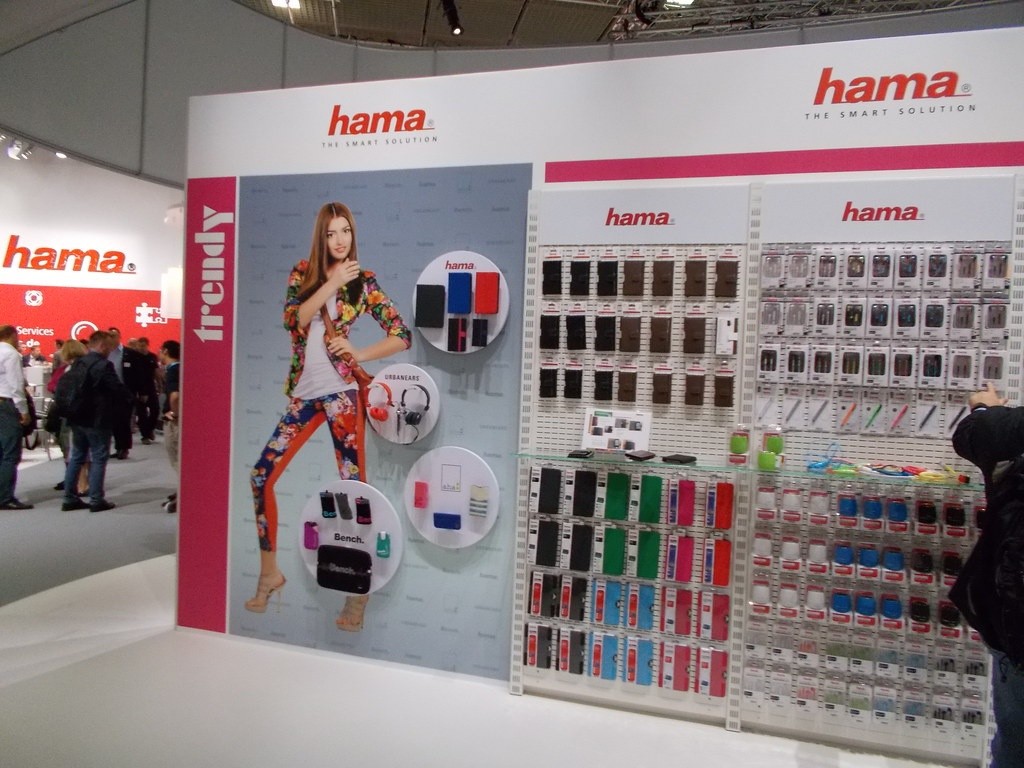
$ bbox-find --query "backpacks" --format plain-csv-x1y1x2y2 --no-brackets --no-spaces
46,358,106,416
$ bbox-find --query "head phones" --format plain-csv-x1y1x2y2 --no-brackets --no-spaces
365,382,392,422
400,384,430,425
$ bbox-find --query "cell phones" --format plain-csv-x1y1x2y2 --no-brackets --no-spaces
303,489,390,558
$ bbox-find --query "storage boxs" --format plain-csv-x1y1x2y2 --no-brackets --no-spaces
20,383,56,449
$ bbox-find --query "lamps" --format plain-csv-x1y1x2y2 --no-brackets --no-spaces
21,144,34,159
127,263,135,271
7,139,22,160
441,0,464,35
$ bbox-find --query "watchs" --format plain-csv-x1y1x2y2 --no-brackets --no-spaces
971,402,989,413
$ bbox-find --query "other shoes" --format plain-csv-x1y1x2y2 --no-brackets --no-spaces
117,449,129,459
61,498,90,511
149,430,155,441
0,498,34,509
54,481,65,490
141,438,151,445
109,453,117,458
89,499,115,512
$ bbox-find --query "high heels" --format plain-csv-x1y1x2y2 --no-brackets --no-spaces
245,573,286,613
336,594,369,632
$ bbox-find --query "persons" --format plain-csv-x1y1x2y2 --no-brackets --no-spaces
245,202,412,631
0,324,181,512
948,381,1024,768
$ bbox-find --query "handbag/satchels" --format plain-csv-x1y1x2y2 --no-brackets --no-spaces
351,357,372,387
947,455,1024,659
16,388,39,450
